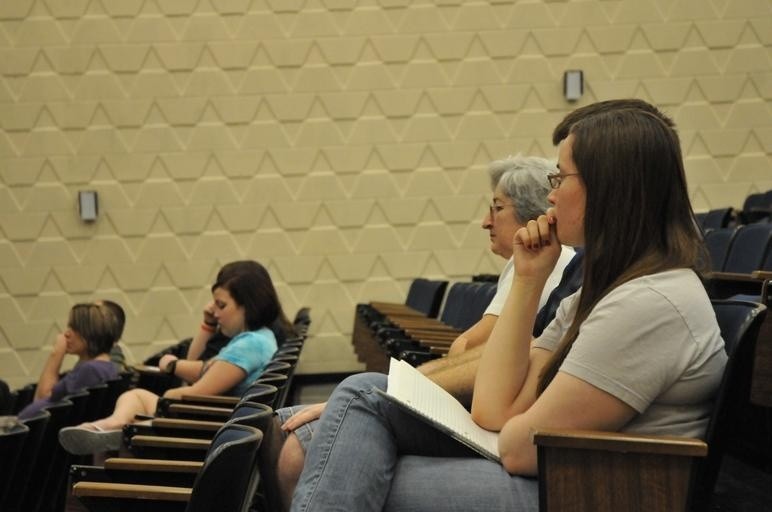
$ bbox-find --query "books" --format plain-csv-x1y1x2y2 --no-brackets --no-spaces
372,354,513,465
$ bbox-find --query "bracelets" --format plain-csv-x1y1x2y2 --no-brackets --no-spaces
200,320,219,332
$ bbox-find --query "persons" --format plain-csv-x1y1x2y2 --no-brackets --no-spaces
292,111,731,512
446,155,584,359
268,98,687,512
91,299,127,380
18,302,121,422
164,260,292,376
54,269,282,460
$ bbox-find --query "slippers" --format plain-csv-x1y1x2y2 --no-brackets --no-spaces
59,423,124,456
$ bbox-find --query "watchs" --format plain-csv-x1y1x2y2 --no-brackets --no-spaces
165,360,176,377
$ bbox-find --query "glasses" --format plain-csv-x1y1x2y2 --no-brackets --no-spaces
547,171,581,188
